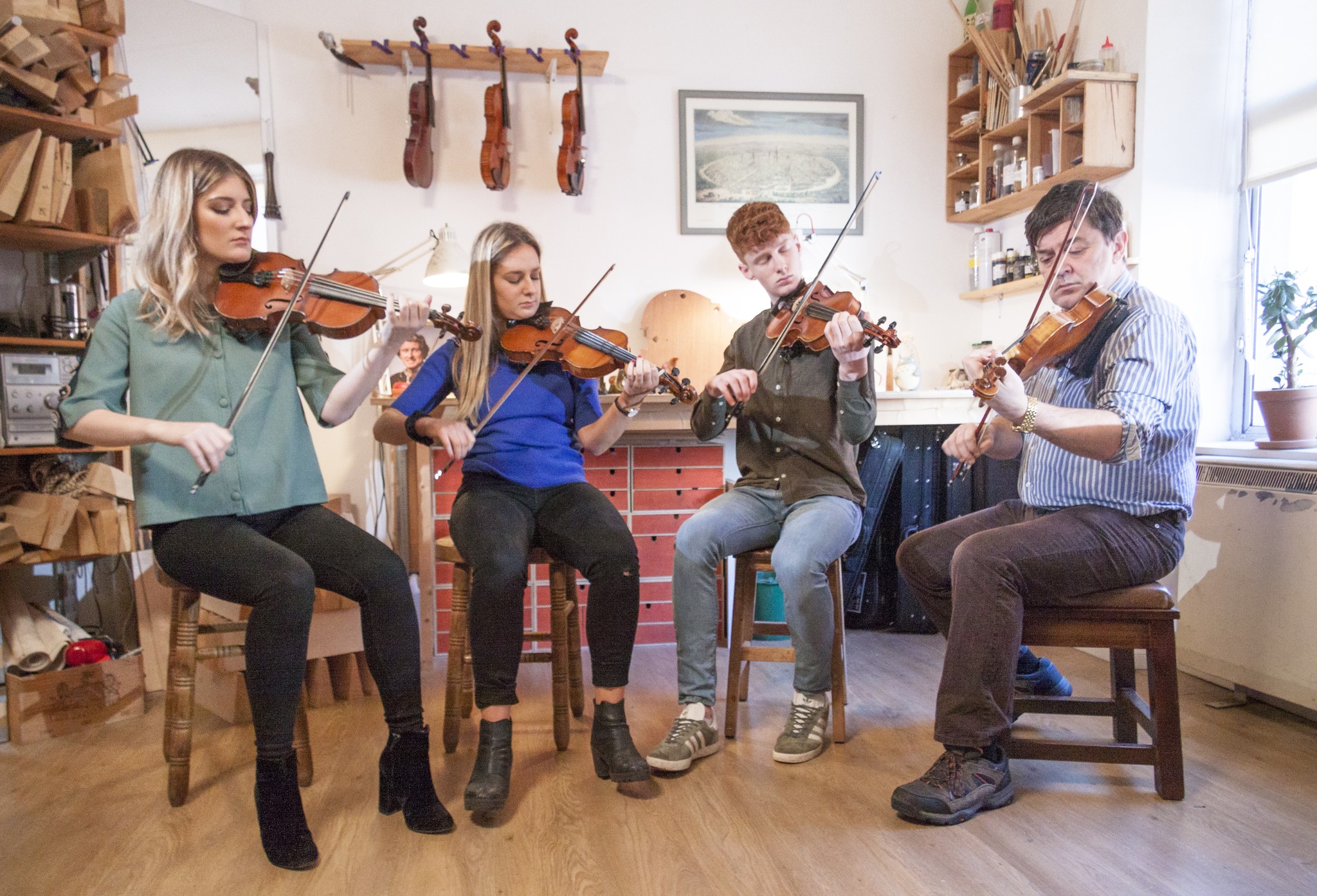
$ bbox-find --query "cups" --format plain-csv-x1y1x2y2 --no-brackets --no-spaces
1008,85,1033,122
957,73,972,98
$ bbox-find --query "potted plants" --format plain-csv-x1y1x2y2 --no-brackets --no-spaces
1251,270,1317,450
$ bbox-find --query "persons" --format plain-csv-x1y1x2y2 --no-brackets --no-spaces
372,220,660,811
889,179,1198,826
59,148,455,871
390,333,429,389
646,202,878,770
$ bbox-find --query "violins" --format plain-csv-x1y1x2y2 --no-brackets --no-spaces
766,281,901,354
479,20,513,192
211,252,484,342
500,306,698,406
403,16,436,188
557,27,588,196
968,285,1120,408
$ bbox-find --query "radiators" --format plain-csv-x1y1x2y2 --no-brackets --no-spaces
1175,464,1317,709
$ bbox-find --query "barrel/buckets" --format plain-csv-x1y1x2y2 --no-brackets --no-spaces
753,571,792,642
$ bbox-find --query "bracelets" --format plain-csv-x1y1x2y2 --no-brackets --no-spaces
405,409,437,446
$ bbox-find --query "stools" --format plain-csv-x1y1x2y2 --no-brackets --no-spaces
1005,578,1184,802
724,547,849,743
435,538,583,751
148,555,316,804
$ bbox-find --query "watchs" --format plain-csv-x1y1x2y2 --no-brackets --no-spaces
614,397,640,417
1010,396,1037,435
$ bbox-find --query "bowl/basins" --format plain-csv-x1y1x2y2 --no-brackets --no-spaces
1076,59,1105,72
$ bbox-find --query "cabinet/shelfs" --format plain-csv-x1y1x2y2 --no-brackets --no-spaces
430,439,726,657
0,0,139,564
947,30,1137,223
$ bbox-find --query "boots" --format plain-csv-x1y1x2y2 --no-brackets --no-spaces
254,749,318,869
464,717,514,819
379,724,455,835
590,698,650,783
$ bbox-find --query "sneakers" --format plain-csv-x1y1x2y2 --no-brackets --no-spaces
891,744,1014,826
1013,657,1073,722
646,703,720,772
772,689,830,764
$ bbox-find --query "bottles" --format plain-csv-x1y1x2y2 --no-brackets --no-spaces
985,136,1027,203
954,191,969,214
1100,36,1114,72
971,341,993,351
968,226,984,291
991,248,1040,286
1032,166,1044,185
979,229,1000,289
954,153,967,170
969,181,979,209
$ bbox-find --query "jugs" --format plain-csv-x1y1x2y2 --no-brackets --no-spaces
46,282,79,340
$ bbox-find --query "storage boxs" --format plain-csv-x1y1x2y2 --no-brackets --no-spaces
5,649,145,746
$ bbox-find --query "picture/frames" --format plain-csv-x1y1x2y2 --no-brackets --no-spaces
677,89,864,234
370,318,457,404
